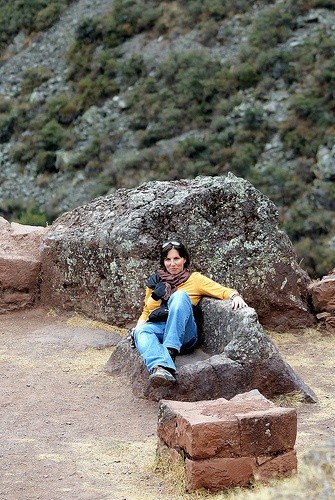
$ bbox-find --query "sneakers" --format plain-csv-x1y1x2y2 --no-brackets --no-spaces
149,367,176,387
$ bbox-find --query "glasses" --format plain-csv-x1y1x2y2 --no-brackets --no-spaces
161,240,181,248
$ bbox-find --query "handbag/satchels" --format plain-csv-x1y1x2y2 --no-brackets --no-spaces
148,307,168,322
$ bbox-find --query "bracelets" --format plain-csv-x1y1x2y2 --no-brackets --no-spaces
231,294,243,300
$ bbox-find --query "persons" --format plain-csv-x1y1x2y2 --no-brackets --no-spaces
128,241,248,389
